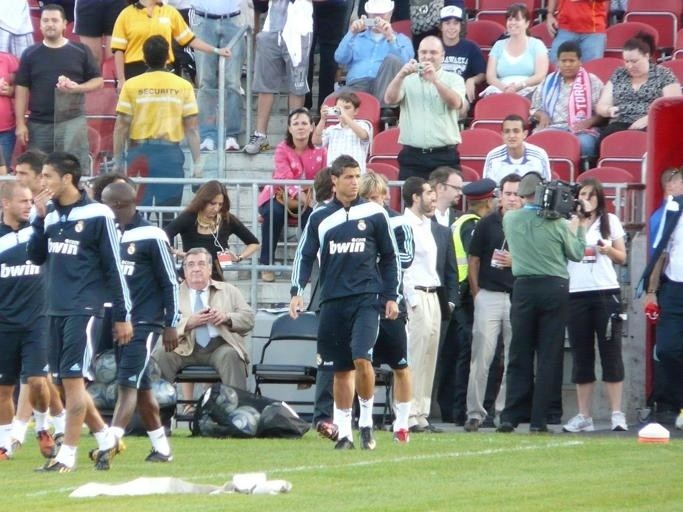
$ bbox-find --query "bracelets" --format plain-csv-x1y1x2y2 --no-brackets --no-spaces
236,253,243,263
211,47,219,55
645,288,658,295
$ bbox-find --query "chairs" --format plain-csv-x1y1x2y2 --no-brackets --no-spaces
353,366,394,429
176,364,220,431
251,314,320,418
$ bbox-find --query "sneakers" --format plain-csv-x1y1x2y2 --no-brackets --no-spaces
334,437,354,449
200,137,215,151
358,426,376,450
675,408,683,430
33,458,72,473
610,413,628,431
635,407,655,423
145,446,173,463
88,434,126,471
53,432,65,446
35,422,57,458
392,428,409,443
10,437,21,450
225,137,239,150
0,447,10,459
242,134,270,154
563,414,594,433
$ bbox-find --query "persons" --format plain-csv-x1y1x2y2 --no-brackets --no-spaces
0,0,35,62
27,150,134,473
528,41,607,170
642,194,683,431
288,155,401,449
188,0,249,151
0,178,59,462
110,0,231,101
112,34,203,246
71,0,130,69
0,50,19,173
544,0,612,67
646,168,680,257
148,247,256,391
669,170,683,197
398,175,443,435
15,4,104,177
435,177,497,426
357,168,415,444
485,4,549,98
482,114,551,198
497,171,586,433
241,0,315,156
462,173,526,430
11,151,67,448
383,35,466,181
562,177,628,433
438,4,486,112
0,145,8,176
162,179,260,283
408,0,465,65
426,167,466,227
312,92,374,181
258,107,328,282
89,178,181,465
92,172,127,205
333,0,415,115
422,181,460,433
307,167,335,427
594,31,683,162
303,0,355,114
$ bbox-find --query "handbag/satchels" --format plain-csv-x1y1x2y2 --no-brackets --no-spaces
190,383,309,438
275,185,314,218
408,0,444,36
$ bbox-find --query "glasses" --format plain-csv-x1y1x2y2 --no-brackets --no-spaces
288,106,308,116
668,167,679,182
442,183,461,191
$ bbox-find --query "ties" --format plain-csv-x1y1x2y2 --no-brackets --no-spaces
194,290,211,348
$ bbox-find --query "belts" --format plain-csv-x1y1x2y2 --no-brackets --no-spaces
408,147,447,154
132,139,173,145
195,10,240,19
414,286,438,293
516,275,544,280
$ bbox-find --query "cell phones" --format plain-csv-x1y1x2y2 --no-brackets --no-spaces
615,106,631,116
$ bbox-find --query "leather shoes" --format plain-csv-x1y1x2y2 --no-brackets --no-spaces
464,419,479,432
390,425,431,432
262,271,274,282
425,420,443,432
496,421,513,432
656,410,676,424
529,425,554,433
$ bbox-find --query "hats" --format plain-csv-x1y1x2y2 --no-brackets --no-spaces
439,5,463,21
518,174,540,196
462,178,497,200
364,0,394,14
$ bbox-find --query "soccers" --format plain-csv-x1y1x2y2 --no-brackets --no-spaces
94,352,117,383
197,411,225,437
229,405,260,436
88,382,107,409
261,402,282,426
106,379,118,409
204,384,238,414
152,379,176,408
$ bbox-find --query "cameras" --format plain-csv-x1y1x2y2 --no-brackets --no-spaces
413,62,424,72
326,107,341,117
364,19,378,27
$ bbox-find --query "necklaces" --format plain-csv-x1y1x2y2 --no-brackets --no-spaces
197,213,220,230
